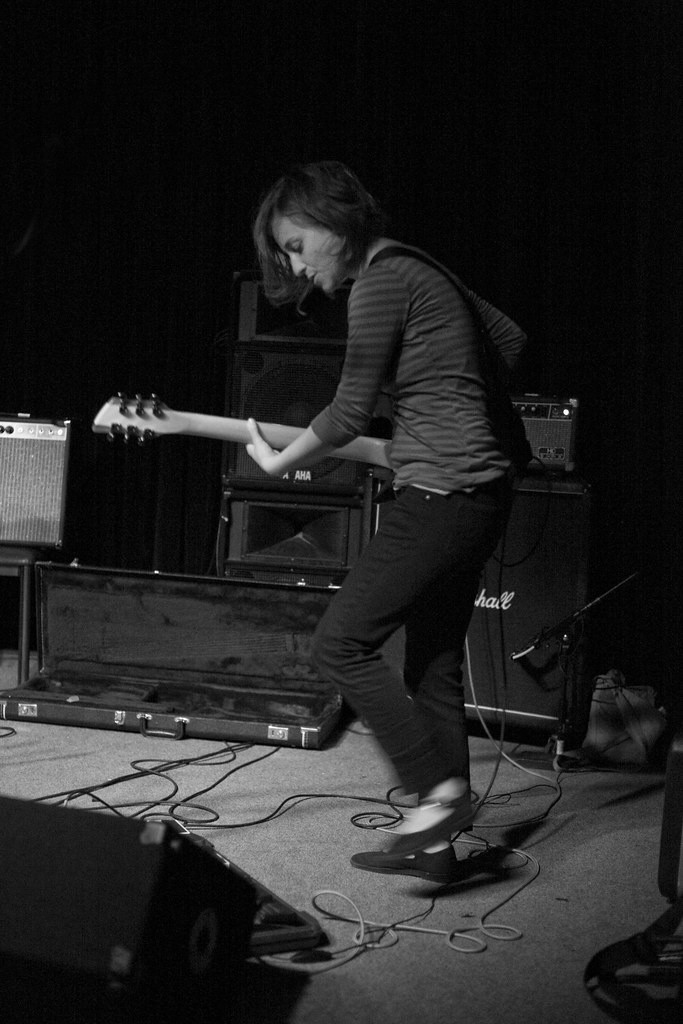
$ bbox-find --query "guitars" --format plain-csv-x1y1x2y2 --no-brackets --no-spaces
88,389,394,482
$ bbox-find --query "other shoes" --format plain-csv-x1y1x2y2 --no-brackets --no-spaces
374,789,475,862
349,842,460,884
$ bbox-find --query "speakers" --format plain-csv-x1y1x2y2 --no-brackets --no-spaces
218,271,383,589
0,412,75,551
0,793,326,1023
459,478,612,730
506,393,579,474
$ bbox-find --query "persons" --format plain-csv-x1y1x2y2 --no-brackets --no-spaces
240,158,535,885
